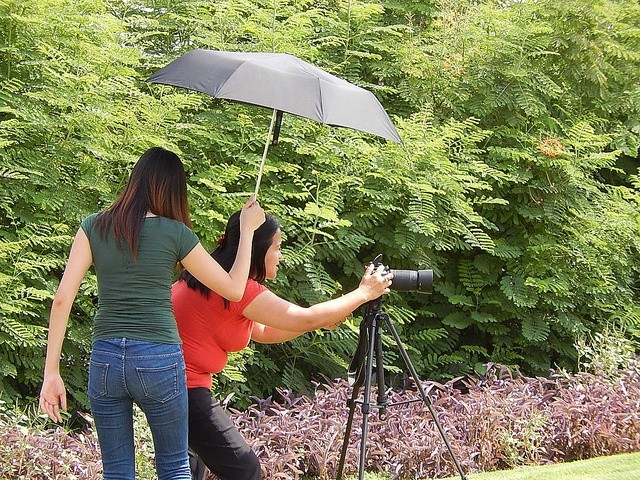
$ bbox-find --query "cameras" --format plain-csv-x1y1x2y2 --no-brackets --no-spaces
365,254,433,300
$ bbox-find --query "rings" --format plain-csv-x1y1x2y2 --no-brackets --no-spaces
383,277,389,282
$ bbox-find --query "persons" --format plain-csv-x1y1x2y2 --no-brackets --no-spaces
168,208,395,480
36,143,267,480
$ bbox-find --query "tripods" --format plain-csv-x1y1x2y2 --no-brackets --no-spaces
336,301,467,480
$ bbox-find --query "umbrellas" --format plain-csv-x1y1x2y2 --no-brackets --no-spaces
143,47,403,206
